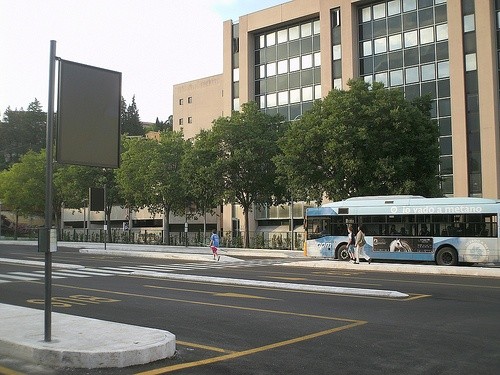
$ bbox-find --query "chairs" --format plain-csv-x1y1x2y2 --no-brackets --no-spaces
322,225,489,237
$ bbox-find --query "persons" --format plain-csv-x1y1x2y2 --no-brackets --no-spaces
400,227,406,236
390,225,397,236
320,225,327,235
210,229,220,263
447,225,454,236
345,227,356,264
419,225,428,236
479,223,489,236
354,226,371,265
457,223,463,232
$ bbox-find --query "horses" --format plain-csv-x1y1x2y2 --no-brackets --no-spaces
390,239,411,252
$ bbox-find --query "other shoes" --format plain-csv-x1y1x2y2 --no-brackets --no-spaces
353,261,360,264
217,255,220,261
350,258,356,262
213,258,217,260
368,257,373,264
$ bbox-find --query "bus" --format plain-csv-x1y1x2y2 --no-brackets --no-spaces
303,194,500,266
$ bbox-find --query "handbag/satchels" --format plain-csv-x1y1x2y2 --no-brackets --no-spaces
358,239,366,247
